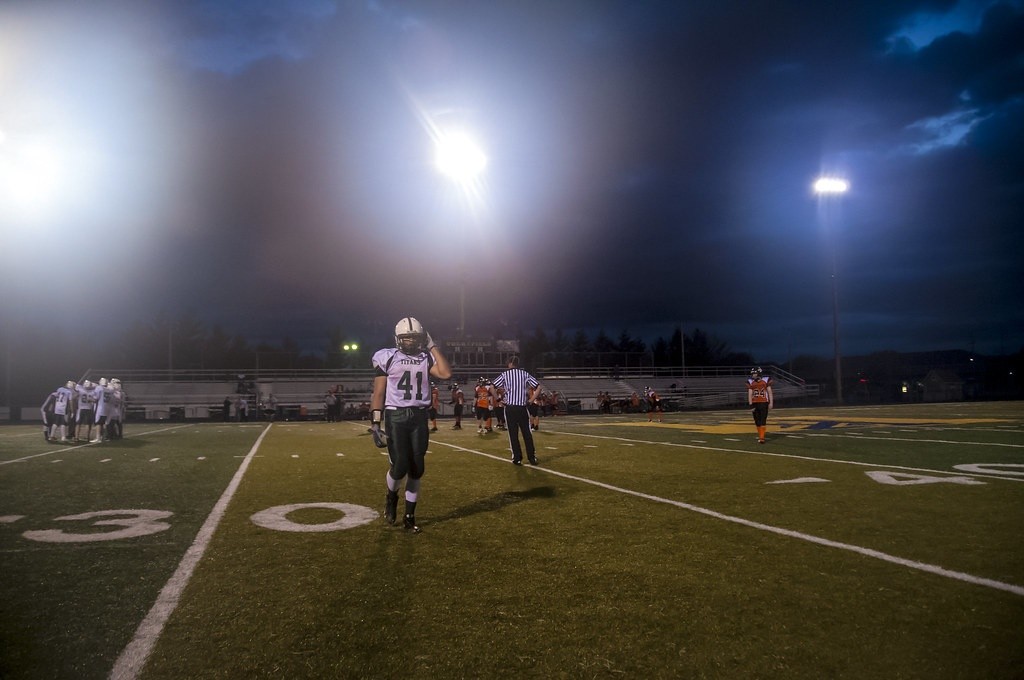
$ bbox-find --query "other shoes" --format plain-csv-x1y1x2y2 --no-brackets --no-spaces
756,436,759,440
477,428,482,433
484,424,504,433
45,435,122,443
531,427,537,431
530,458,538,464
453,424,461,429
431,427,437,431
649,419,652,422
658,419,661,422
514,460,521,465
758,439,765,442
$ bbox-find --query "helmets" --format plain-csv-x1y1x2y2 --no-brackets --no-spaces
751,366,763,377
477,377,485,385
66,377,121,391
644,386,649,392
430,382,435,387
452,383,458,390
485,379,491,385
394,317,424,356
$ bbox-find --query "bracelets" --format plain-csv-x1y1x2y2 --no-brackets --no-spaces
497,398,501,402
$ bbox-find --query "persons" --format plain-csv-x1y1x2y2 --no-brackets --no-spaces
429,381,438,431
262,393,277,422
631,391,639,410
745,366,773,443
239,398,248,421
603,391,611,414
224,396,233,422
326,389,337,423
472,376,540,433
644,386,662,423
367,317,452,534
490,356,541,465
40,378,126,442
449,382,463,428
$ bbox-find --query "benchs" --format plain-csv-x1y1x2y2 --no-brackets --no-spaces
303,408,326,420
125,407,145,423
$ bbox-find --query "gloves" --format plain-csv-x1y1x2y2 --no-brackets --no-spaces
750,404,755,411
472,406,475,412
424,330,436,351
372,423,389,447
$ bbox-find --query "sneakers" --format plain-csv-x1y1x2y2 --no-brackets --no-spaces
383,495,398,524
403,514,421,533
